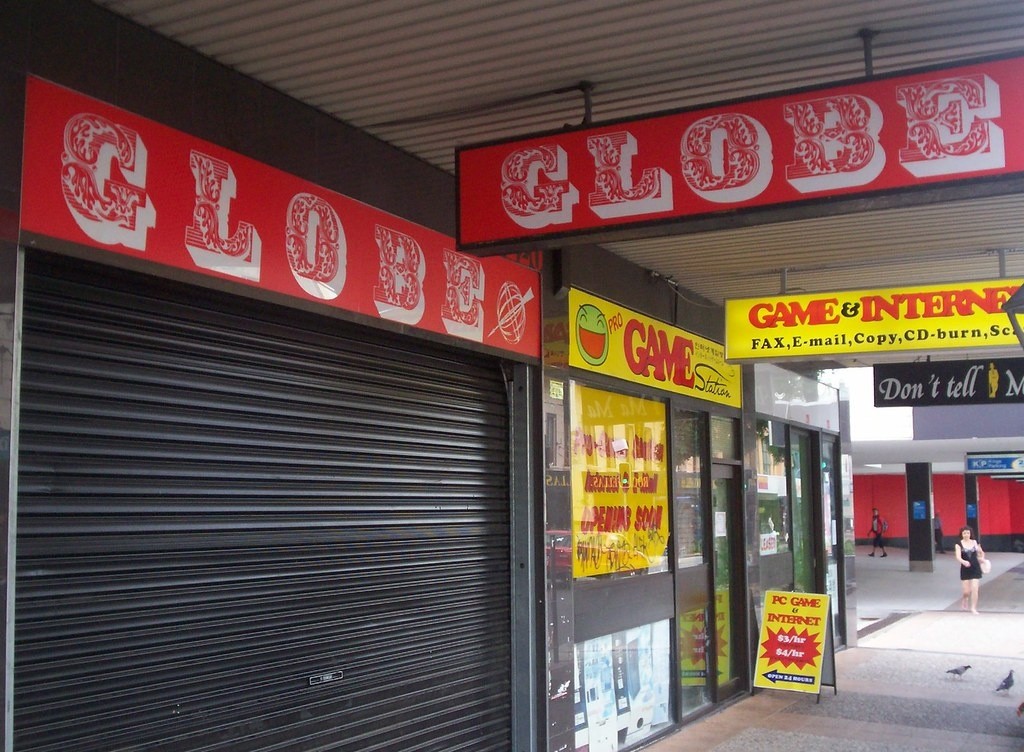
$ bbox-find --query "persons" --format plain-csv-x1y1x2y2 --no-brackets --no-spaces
954,526,985,615
867,507,887,557
934,513,946,554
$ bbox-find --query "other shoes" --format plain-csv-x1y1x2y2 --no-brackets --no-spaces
941,551,945,553
868,552,874,556
962,598,969,610
880,553,887,557
971,609,979,615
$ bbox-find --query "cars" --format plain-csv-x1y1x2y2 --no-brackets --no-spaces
546,530,648,581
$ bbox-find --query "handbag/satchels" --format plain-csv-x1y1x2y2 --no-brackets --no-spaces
980,558,991,574
